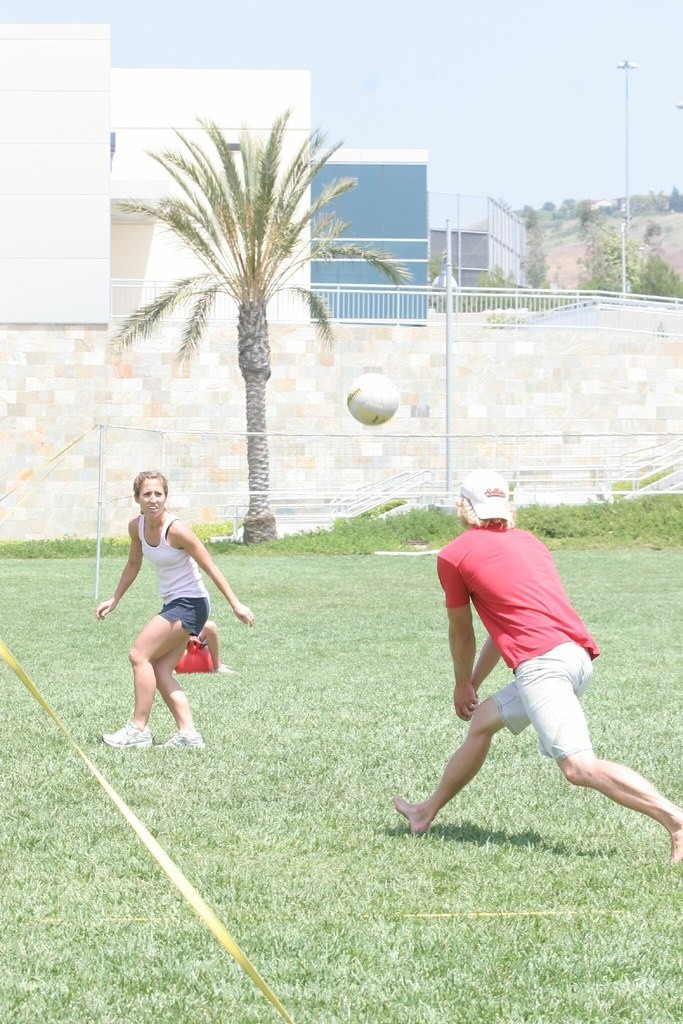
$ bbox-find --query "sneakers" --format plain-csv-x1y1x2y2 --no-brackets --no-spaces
102,722,152,747
155,731,205,749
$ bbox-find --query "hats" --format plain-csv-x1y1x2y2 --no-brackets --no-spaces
462,469,510,522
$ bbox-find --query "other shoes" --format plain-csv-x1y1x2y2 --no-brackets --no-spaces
212,664,235,675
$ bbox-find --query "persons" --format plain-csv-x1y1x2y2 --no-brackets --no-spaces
391,468,683,869
95,468,255,752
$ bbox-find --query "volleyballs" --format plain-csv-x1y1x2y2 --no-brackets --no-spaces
348,371,399,427
